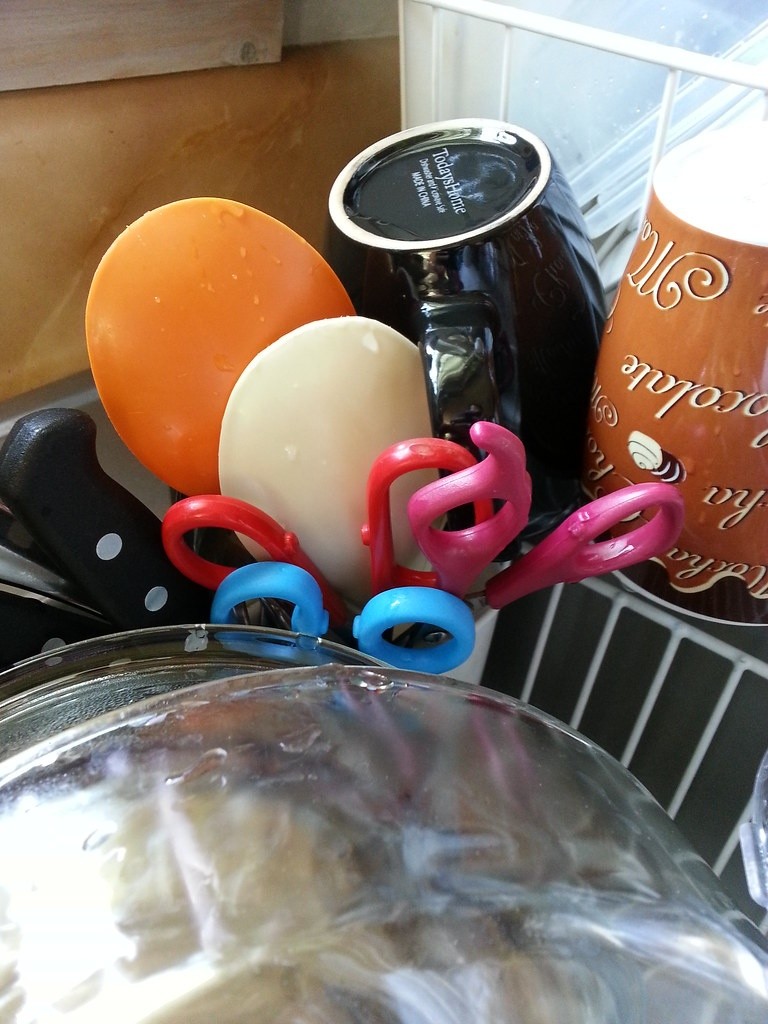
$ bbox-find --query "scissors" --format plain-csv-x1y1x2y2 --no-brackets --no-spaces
160,422,686,676
